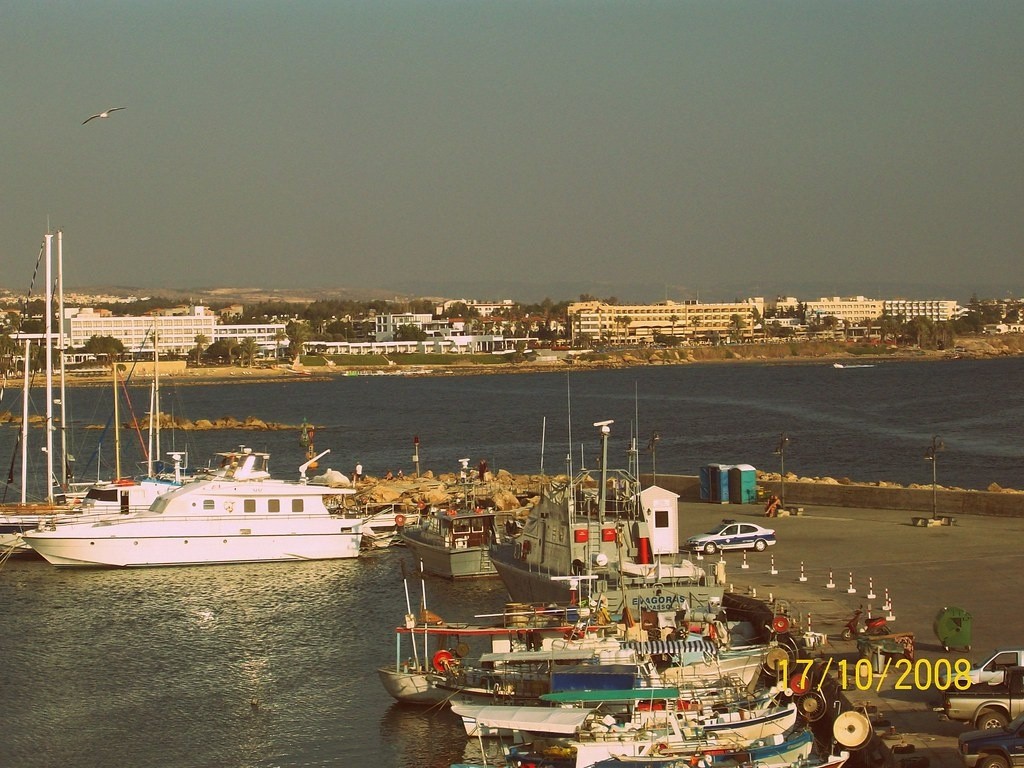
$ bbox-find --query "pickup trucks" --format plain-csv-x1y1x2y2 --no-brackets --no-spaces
937,665,1024,730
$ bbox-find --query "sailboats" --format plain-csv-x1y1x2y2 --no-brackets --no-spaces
0,228,210,559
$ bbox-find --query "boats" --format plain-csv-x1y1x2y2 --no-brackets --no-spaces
832,362,876,372
394,458,504,578
375,585,857,767
489,366,706,603
351,501,445,531
19,444,366,568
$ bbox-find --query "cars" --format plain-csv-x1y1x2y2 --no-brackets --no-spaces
683,519,777,555
958,710,1024,768
941,646,1024,686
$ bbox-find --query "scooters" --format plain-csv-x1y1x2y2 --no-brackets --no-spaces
840,605,891,642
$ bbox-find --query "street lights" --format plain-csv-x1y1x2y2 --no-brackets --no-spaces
772,430,789,508
646,429,660,486
924,433,945,518
